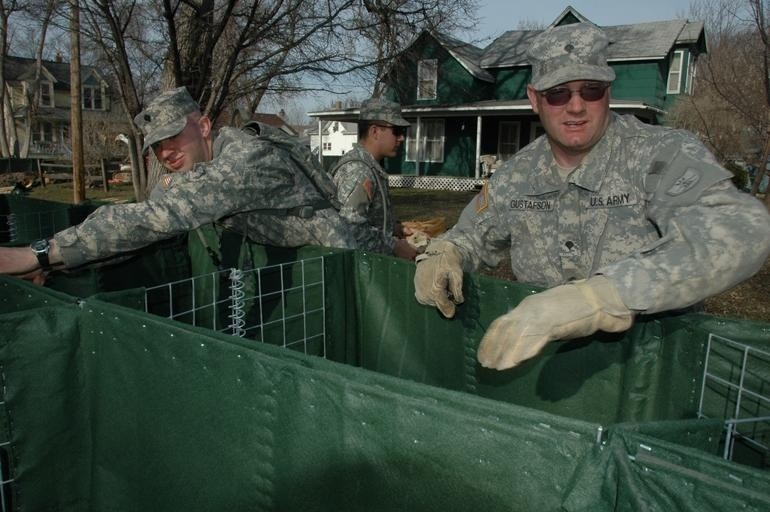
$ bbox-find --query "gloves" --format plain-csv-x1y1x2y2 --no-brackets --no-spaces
413,234,464,319
476,274,634,371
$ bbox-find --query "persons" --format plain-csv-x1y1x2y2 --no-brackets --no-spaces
410,20,770,375
1,83,357,289
328,93,423,259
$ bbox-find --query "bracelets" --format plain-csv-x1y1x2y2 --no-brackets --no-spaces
29,236,53,274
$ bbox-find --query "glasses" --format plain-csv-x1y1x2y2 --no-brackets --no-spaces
367,123,408,137
538,84,612,107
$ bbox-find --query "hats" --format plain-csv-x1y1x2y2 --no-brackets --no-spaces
356,98,412,129
525,22,616,94
132,85,201,158
